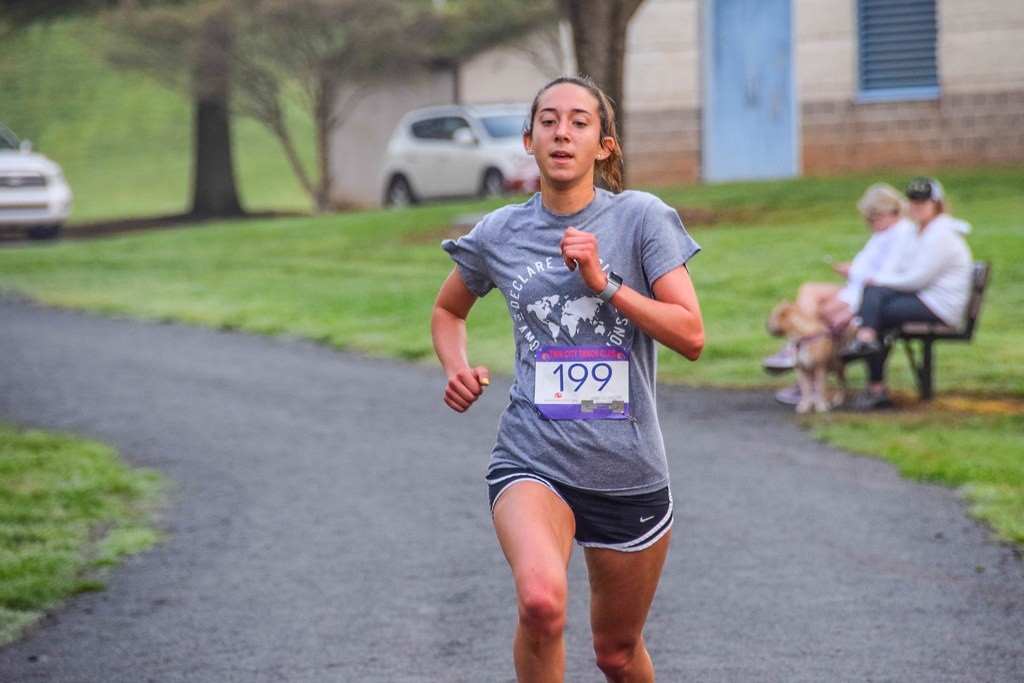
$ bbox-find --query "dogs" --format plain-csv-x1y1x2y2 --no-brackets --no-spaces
765,303,834,414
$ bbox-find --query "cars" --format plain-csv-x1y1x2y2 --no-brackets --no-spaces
0,125,72,245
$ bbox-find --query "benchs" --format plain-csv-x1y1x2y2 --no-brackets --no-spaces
900,257,995,403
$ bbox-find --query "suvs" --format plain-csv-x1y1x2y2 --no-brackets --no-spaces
377,99,545,206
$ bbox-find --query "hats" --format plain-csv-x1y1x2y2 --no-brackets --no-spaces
905,176,944,204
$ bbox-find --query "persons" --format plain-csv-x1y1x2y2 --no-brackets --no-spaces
831,175,976,415
762,181,921,408
429,74,706,683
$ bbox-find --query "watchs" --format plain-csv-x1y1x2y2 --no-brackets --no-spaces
595,270,623,302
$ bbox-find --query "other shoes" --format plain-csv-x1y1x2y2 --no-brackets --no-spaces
763,349,796,374
772,381,822,406
842,388,895,412
